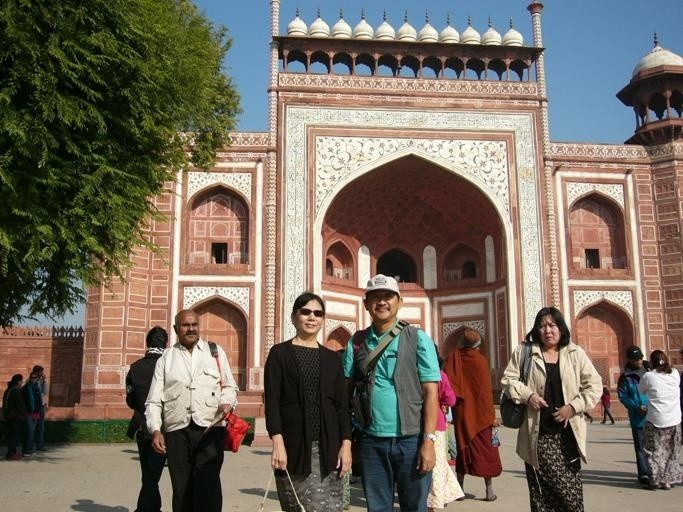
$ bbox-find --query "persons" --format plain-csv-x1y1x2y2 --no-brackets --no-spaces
602,388,614,426
126,325,172,511
33,365,48,451
445,325,501,502
639,350,683,489
3,374,28,461
21,372,42,457
617,345,652,486
145,310,240,512
500,306,604,512
342,274,443,512
267,292,354,512
425,343,465,511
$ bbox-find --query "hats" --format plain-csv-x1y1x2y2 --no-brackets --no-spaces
364,274,400,296
627,346,643,358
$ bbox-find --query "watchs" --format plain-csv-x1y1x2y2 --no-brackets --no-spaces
424,431,438,442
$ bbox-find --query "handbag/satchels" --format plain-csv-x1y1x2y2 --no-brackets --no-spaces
499,378,526,427
491,435,500,448
223,414,251,453
349,382,367,443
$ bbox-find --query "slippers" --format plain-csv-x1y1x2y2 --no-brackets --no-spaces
663,484,670,490
486,495,496,501
643,483,658,490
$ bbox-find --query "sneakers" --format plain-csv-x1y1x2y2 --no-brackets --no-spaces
21,453,31,457
36,448,47,451
9,455,21,459
638,476,649,483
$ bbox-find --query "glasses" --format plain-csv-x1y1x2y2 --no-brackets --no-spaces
297,308,324,317
30,377,36,379
